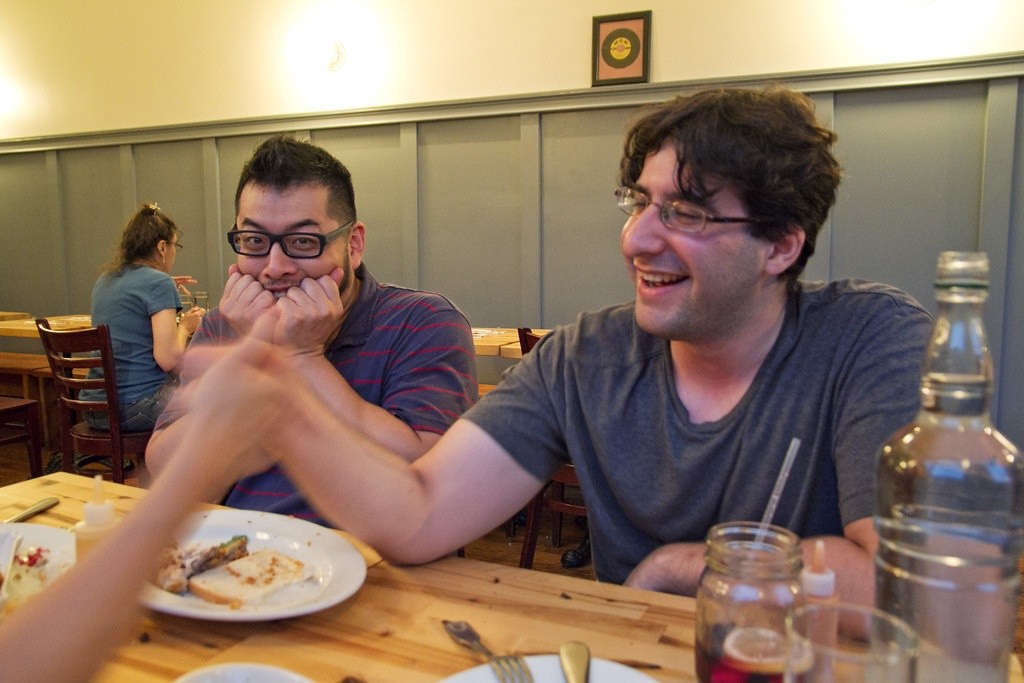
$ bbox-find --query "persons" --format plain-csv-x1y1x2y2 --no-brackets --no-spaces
1,338,284,683
80,205,206,430
145,133,477,528
191,89,1004,637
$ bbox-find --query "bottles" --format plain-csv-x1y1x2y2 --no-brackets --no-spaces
875,249,1024,683
74,475,122,568
793,539,840,683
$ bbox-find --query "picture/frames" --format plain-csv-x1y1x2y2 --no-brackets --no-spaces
591,10,652,88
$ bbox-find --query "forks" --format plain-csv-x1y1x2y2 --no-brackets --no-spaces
442,619,535,683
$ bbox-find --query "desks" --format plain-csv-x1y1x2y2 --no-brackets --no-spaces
0,472,1024,683
0,311,557,359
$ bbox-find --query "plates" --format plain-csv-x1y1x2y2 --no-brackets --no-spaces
135,509,367,622
0,522,76,621
435,653,658,683
173,662,317,683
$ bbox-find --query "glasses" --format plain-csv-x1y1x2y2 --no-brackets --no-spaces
227,221,353,259
153,240,184,252
614,185,763,237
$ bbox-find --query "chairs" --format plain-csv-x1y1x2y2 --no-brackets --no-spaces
0,397,43,478
33,318,154,485
515,327,590,569
0,352,498,452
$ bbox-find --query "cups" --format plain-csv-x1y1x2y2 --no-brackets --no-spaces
193,291,208,310
178,294,193,312
783,600,918,683
694,521,787,683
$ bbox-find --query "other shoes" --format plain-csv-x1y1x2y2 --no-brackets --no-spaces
563,537,590,566
